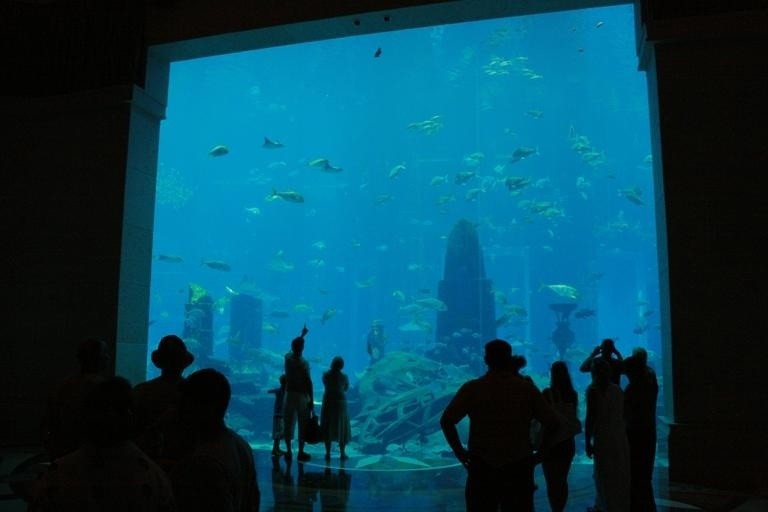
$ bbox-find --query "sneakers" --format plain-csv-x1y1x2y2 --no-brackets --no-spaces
298,453,312,461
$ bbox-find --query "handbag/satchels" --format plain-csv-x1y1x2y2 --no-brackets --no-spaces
304,408,324,445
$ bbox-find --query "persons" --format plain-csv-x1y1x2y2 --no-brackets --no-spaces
284,323,316,461
266,372,286,455
320,355,350,460
436,338,659,512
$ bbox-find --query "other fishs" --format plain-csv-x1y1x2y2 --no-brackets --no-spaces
149,13,662,384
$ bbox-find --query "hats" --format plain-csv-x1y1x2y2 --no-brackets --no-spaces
151,335,194,369
